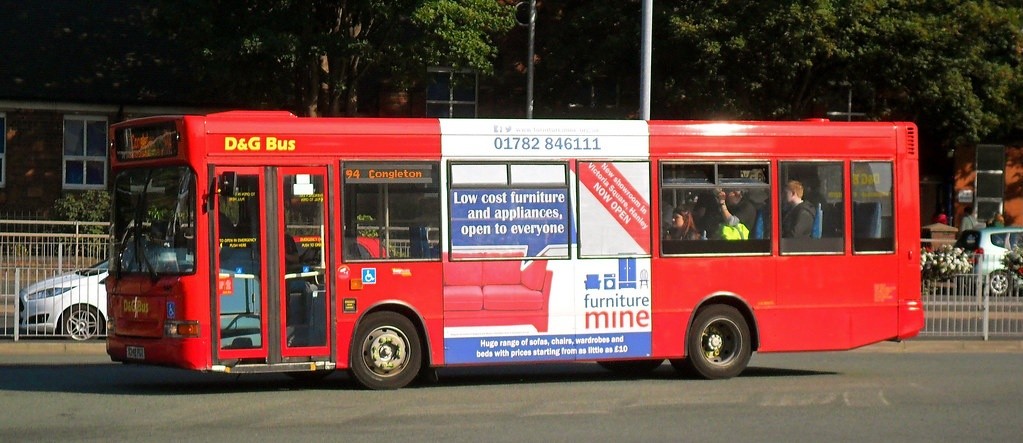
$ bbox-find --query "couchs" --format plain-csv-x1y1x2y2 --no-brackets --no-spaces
442,251,553,333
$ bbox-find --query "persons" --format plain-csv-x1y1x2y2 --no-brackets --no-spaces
959,205,977,240
216,202,238,246
985,209,1006,229
283,215,309,294
927,199,949,225
782,181,816,239
662,166,779,248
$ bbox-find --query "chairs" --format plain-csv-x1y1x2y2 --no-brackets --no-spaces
408,224,439,258
749,202,882,245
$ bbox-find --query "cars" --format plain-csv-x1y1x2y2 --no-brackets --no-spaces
952,226,1023,298
16,255,111,340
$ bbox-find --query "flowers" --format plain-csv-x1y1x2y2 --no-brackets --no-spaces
998,233,1023,289
918,242,973,289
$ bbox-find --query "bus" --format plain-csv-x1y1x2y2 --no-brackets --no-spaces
103,109,924,390
